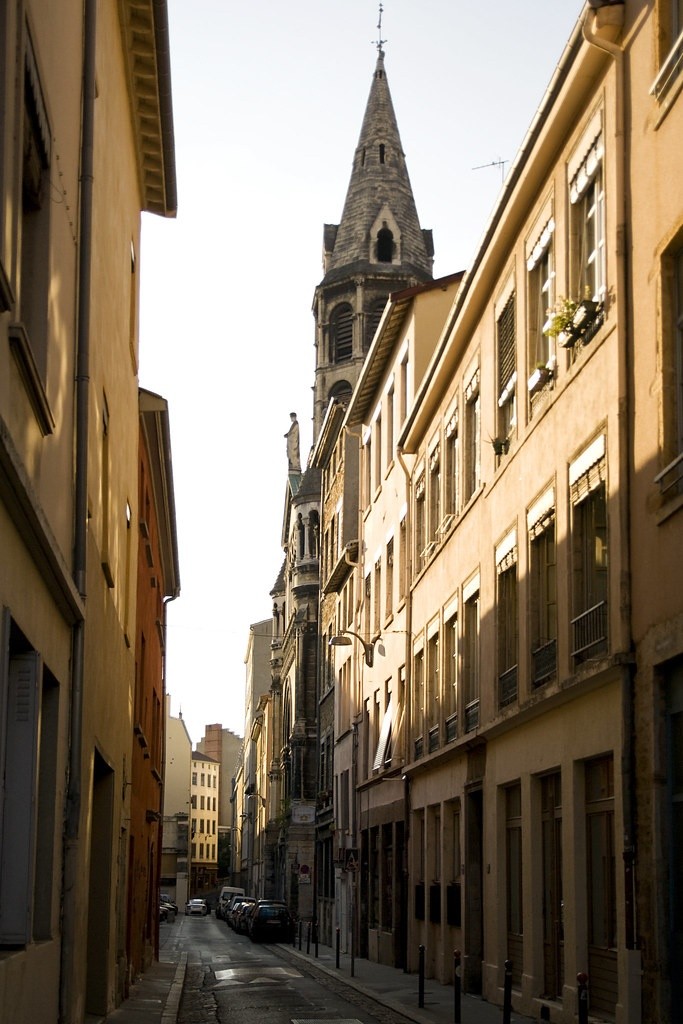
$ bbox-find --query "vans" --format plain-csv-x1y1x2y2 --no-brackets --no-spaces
217,887,245,919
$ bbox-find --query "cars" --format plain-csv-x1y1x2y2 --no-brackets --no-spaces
185,899,208,916
194,898,211,914
223,895,295,942
